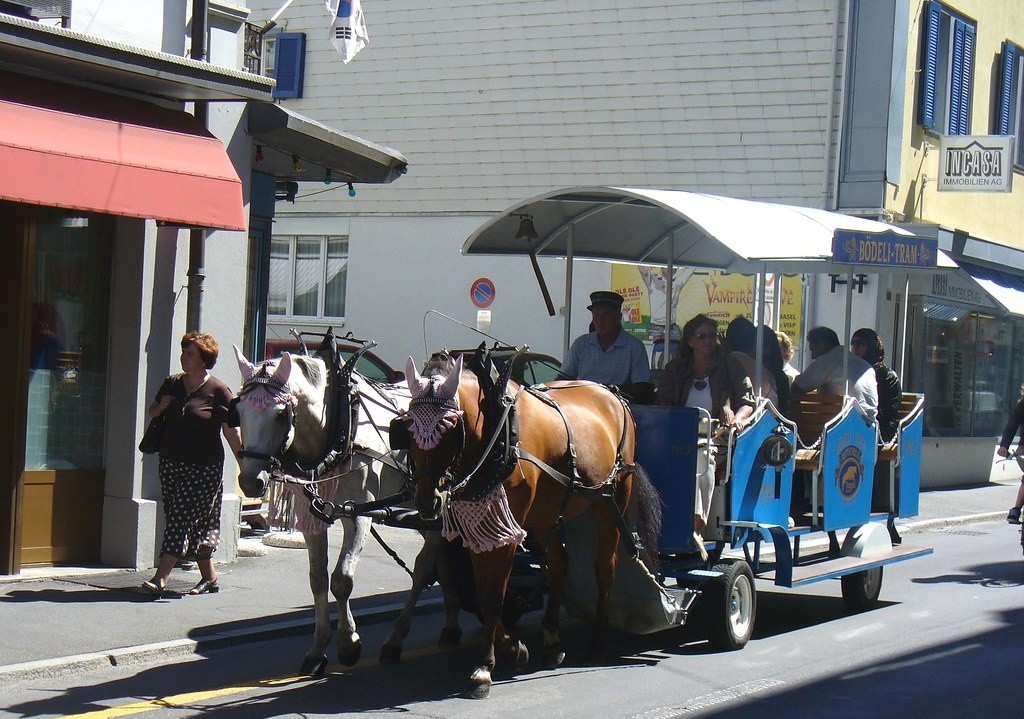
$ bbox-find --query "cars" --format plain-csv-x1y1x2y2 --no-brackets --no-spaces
263,337,407,385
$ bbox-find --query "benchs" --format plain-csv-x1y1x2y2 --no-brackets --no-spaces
702,389,923,541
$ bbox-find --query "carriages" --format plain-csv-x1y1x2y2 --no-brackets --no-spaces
224,180,964,703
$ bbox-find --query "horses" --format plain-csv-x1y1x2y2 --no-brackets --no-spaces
228,343,666,698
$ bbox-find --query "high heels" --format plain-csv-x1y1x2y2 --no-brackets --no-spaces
190,578,219,594
142,576,165,600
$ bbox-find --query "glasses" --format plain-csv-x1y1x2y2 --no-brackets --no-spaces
692,332,718,340
852,339,865,349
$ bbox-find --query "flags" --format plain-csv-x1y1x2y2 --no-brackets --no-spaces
324,0,369,64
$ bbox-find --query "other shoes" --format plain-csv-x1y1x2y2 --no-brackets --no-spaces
1007,507,1022,523
788,514,797,528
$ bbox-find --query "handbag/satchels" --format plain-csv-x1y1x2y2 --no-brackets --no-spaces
139,373,180,454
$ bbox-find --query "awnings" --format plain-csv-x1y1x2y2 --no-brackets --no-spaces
920,249,1024,318
0,68,247,232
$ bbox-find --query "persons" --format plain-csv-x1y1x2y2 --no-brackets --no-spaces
654,314,903,549
555,291,653,404
141,332,245,599
997,388,1024,525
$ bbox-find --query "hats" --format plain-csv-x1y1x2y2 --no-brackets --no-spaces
586,291,625,311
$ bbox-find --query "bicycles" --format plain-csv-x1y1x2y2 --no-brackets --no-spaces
995,449,1024,562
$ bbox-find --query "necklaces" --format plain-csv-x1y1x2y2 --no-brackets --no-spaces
694,373,709,390
